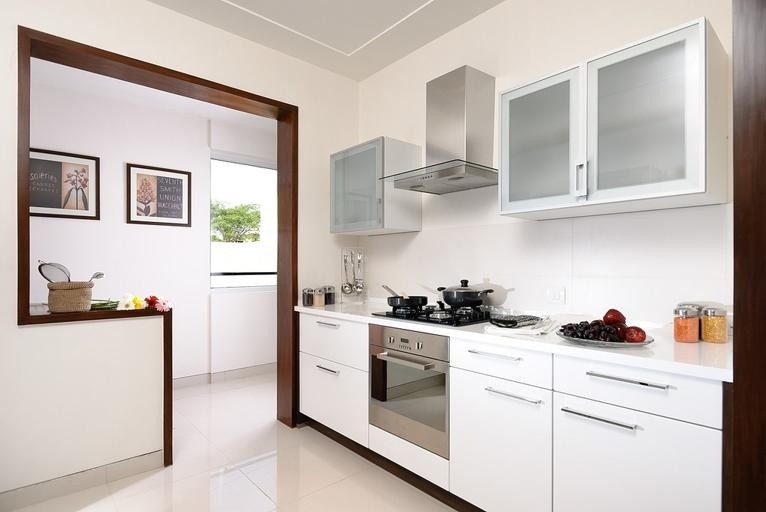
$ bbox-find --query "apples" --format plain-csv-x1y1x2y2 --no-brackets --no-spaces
624,326,646,343
603,308,626,325
612,323,627,342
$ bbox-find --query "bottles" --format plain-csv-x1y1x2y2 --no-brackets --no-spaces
325,286,335,304
678,302,700,320
313,288,325,307
303,288,313,306
701,307,728,343
674,308,700,343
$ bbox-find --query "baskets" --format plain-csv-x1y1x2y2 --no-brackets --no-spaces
48,283,94,312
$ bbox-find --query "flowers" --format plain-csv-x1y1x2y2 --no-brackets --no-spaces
129,295,174,313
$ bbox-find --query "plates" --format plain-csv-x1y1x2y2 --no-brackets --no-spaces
556,330,654,349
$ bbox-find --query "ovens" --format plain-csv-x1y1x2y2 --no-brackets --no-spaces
369,323,450,460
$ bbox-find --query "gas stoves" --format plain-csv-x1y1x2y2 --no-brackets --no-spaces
371,307,503,327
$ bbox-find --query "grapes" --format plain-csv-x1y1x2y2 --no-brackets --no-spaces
559,319,618,342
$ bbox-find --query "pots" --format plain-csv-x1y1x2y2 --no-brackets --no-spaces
437,279,494,306
382,284,428,306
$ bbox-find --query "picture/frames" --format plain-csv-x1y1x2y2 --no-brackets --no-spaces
30,148,100,222
127,163,192,228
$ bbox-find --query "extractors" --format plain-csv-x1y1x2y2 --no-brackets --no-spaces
378,65,498,196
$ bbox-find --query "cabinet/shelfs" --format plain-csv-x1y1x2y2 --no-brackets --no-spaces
295,307,368,458
498,16,730,219
448,329,556,512
554,354,734,512
329,136,423,238
